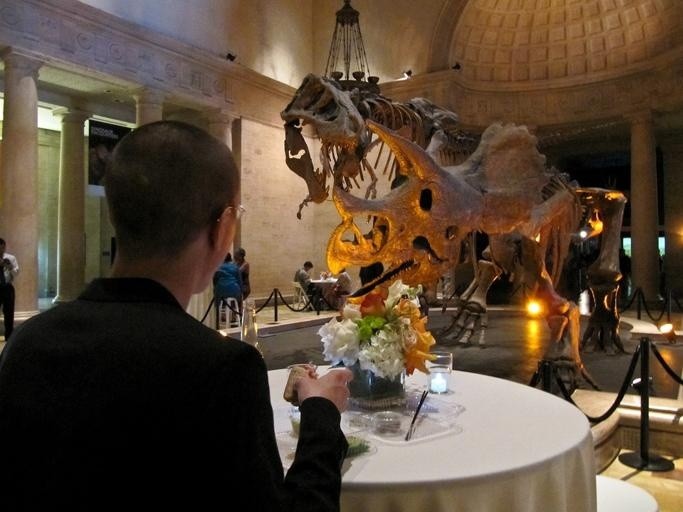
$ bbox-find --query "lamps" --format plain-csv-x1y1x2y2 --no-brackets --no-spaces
403,70,412,78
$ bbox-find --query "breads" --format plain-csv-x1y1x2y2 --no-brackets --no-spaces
284,363,318,406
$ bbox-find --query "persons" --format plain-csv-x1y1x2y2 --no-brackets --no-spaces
325,268,353,312
546,241,666,308
293,260,323,311
221,245,252,328
0,119,355,511
214,252,242,329
0,236,23,340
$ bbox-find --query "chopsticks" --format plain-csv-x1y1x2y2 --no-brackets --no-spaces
404,391,431,442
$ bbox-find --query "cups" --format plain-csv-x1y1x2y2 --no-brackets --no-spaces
427,364,451,395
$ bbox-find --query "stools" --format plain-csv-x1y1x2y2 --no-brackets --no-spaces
218,297,240,328
337,293,353,309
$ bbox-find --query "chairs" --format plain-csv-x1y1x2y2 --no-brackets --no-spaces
595,474,658,512
290,281,315,311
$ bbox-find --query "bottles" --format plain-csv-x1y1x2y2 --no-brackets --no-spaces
241,296,264,362
